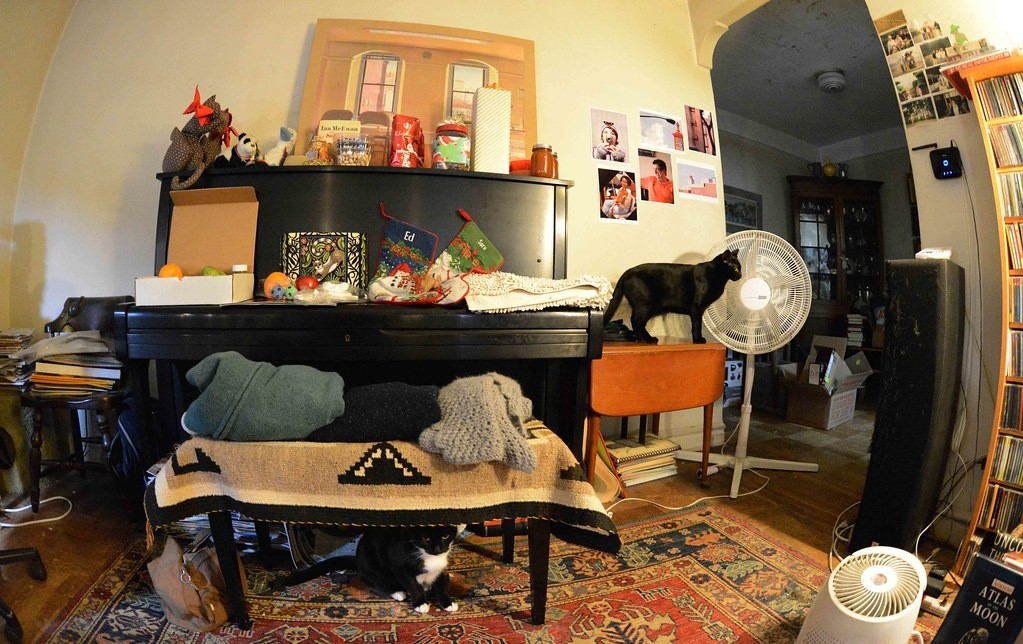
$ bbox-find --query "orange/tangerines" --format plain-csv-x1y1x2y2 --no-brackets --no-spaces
265,272,292,299
159,264,182,280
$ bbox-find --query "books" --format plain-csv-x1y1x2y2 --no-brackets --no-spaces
848,314,865,346
603,429,681,487
146,444,258,542
0,328,122,395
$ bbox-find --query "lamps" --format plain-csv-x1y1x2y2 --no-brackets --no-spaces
815,69,846,93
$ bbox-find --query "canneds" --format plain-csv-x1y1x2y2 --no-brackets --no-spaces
531,144,559,179
431,124,471,170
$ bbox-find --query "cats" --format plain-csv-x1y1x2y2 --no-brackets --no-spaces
267,524,458,614
603,248,742,345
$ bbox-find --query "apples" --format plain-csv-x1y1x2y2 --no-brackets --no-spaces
203,266,227,275
296,277,318,291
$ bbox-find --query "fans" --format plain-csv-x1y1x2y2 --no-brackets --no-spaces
672,231,818,498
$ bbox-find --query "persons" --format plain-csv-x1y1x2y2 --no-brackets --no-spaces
600,175,632,221
887,20,970,124
591,119,626,163
642,158,674,203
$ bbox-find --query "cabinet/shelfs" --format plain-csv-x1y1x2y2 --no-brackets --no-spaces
953,50,1023,578
786,174,884,317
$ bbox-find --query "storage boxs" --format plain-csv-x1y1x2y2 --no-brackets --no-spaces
724,335,873,431
134,187,260,306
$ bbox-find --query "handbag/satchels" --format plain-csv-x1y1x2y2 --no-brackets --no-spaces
147,528,248,632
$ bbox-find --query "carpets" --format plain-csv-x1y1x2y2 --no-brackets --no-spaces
33,502,942,644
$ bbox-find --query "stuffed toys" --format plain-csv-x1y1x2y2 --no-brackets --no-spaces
161,95,239,190
259,127,299,166
214,133,259,168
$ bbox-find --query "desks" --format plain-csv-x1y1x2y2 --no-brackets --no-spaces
590,336,722,495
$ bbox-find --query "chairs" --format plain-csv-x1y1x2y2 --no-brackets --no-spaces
28,296,131,508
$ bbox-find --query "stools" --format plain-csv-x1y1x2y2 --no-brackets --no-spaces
191,418,552,625
